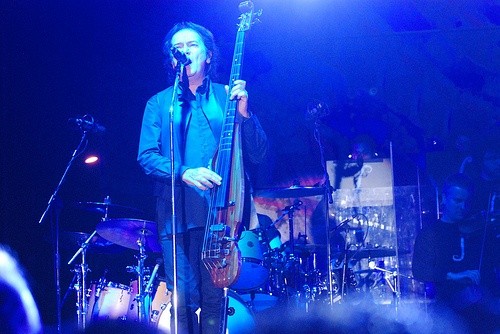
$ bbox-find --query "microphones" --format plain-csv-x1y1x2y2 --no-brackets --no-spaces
337,213,357,229
310,103,322,115
169,43,191,66
75,118,106,135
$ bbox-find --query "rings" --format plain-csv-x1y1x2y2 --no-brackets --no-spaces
471,274,475,278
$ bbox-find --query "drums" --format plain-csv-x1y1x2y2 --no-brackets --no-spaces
273,246,321,291
228,229,270,291
327,257,354,270
127,280,167,322
86,279,135,323
149,289,258,334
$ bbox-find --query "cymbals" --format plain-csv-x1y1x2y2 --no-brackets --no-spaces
96,218,160,255
264,185,326,198
75,202,146,216
347,247,395,258
58,232,114,252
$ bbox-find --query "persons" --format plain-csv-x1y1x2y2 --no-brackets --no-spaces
137,22,267,334
412,174,500,334
249,195,285,257
